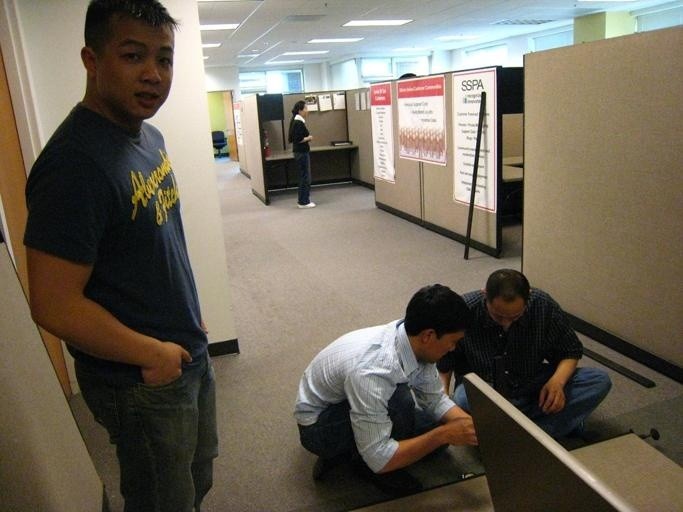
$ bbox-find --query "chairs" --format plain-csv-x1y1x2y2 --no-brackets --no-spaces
212,131,229,158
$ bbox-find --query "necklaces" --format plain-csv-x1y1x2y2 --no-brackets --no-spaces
91,99,108,120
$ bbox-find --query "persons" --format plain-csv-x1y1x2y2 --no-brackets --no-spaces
432,268,612,445
287,101,315,209
20,0,217,508
292,284,479,497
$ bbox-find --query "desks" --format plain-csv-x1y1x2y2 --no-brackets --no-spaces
335,372,683,512
262,140,360,204
501,147,523,221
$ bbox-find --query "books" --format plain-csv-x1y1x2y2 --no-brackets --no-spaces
331,141,351,146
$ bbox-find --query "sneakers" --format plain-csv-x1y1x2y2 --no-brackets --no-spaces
381,470,419,496
313,457,331,479
296,200,316,209
